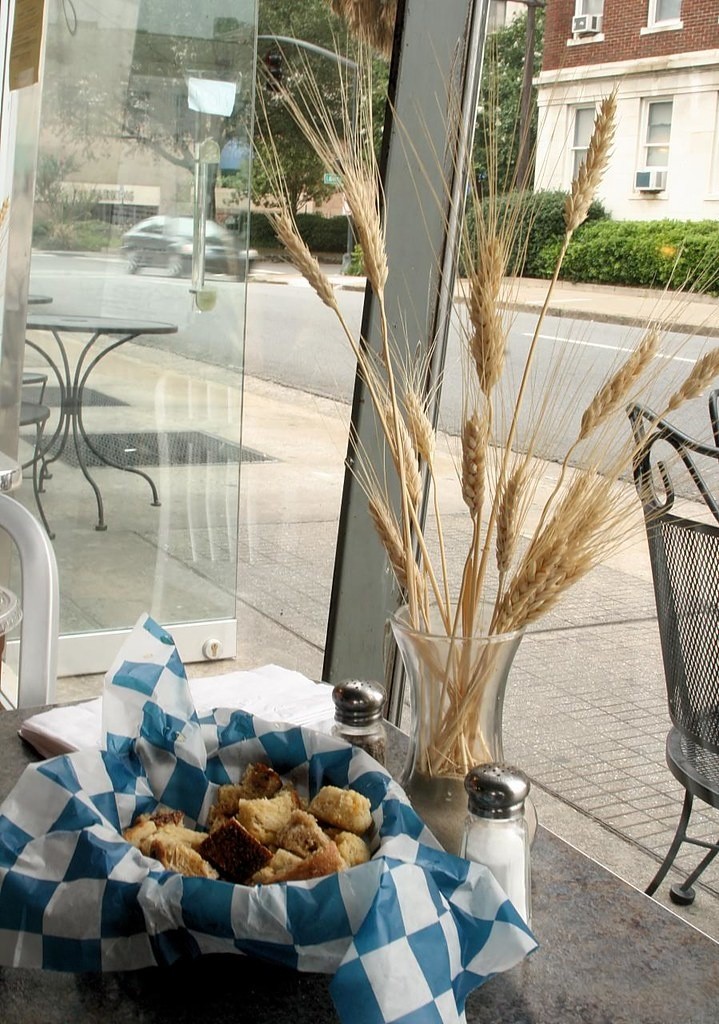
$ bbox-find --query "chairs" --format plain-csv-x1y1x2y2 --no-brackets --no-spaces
20,373,55,540
627,389,719,907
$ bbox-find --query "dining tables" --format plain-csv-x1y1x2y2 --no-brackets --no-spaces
0,681,719,1024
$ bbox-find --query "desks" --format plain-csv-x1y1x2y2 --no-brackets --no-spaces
28,294,52,304
24,313,178,531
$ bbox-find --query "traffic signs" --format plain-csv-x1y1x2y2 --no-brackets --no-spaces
323,173,343,186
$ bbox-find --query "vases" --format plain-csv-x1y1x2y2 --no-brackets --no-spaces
389,597,538,856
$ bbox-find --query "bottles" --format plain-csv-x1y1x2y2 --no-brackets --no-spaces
330,677,390,771
462,762,530,929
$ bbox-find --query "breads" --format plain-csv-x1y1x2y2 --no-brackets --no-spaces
122,761,373,885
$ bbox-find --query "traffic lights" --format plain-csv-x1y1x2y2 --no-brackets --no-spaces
215,53,236,83
333,158,346,176
262,50,285,94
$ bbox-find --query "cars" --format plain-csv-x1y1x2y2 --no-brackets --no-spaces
118,215,260,283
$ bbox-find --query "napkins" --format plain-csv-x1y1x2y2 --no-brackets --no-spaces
17,663,339,761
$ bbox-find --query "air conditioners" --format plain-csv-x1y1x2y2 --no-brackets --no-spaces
634,168,667,191
571,14,600,33
